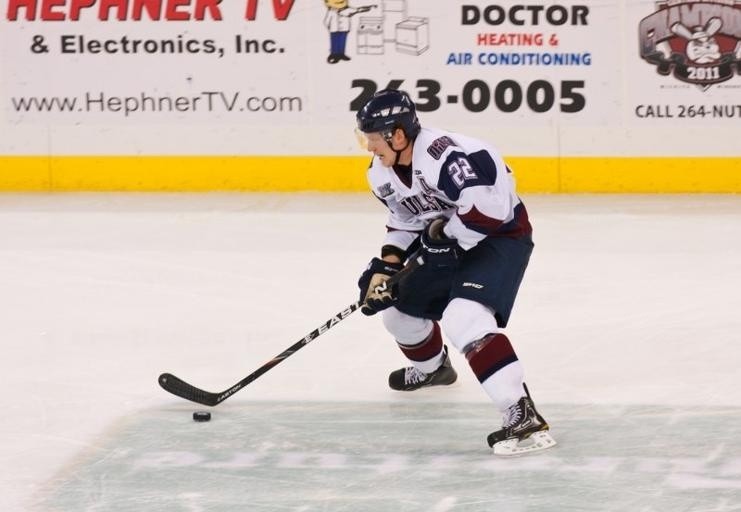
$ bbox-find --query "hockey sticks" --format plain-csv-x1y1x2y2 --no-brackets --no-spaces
158,255,428,406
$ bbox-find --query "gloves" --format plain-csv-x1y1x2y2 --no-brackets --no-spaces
418,215,461,268
357,257,408,315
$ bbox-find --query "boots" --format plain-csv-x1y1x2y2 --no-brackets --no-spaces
388,343,459,392
487,379,551,446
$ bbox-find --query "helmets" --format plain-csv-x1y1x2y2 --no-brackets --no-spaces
356,88,423,140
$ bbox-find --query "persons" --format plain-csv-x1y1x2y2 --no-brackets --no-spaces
357,89,549,449
